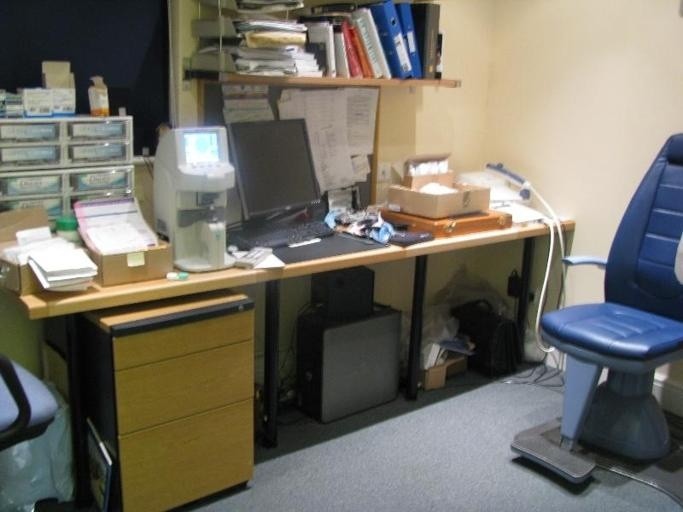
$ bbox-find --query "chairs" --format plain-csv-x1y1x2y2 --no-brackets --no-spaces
511,126,682,489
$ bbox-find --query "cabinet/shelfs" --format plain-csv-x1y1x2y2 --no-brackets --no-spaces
84,286,257,511
1,118,136,230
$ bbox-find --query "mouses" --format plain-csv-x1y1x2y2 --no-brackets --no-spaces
336,212,351,225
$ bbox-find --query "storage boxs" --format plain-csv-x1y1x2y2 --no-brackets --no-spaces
400,150,457,193
378,203,514,243
0,235,93,302
74,222,177,283
383,177,494,220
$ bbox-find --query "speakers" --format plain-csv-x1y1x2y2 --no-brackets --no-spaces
311,268,374,319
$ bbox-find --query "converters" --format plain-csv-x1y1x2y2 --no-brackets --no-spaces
507,277,520,297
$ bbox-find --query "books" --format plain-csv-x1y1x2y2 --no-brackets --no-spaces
304,9,388,80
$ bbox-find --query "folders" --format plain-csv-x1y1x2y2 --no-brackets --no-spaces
358,1,422,79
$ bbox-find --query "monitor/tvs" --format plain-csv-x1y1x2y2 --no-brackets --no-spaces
229,118,318,220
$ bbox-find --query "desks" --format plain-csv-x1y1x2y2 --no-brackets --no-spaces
1,187,580,510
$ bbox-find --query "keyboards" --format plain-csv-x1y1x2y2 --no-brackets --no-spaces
232,221,332,249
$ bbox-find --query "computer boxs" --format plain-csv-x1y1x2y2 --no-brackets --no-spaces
298,305,402,420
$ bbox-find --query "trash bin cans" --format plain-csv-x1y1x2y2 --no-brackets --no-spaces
0,352,76,512
